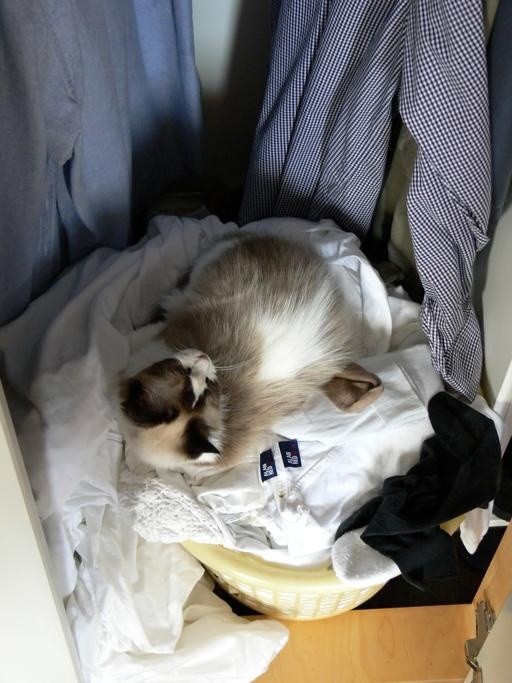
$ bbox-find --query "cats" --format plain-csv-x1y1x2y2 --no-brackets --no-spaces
112,229,352,475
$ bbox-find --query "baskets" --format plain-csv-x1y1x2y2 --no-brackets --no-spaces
181,513,468,624
